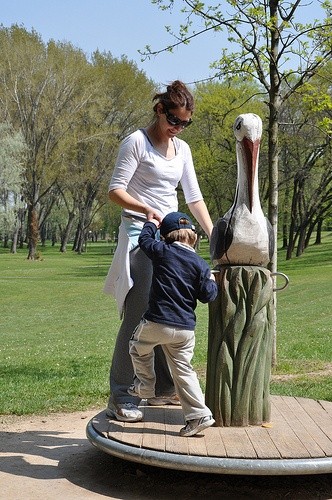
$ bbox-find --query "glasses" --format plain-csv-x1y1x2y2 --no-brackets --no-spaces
164,107,193,128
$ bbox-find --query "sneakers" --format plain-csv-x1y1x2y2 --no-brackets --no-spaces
179,415,215,436
147,389,181,405
127,384,147,398
106,402,143,422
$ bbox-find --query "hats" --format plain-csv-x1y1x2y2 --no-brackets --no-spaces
160,212,196,235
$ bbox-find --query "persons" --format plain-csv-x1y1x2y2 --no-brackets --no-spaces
128,212,218,436
106,79,214,423
